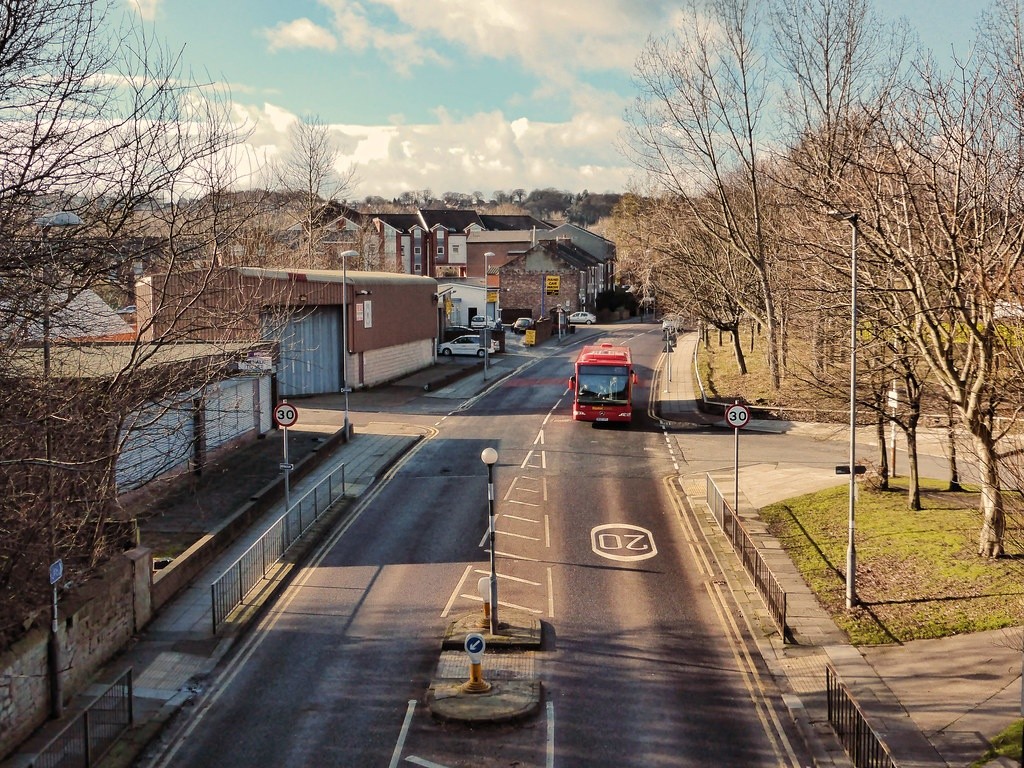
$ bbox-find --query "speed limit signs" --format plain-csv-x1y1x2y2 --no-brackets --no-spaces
725,404,750,428
273,403,299,427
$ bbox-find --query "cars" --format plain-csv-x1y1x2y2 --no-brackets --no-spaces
115,305,136,316
514,318,534,334
436,335,501,358
443,324,473,332
567,311,597,325
662,313,686,332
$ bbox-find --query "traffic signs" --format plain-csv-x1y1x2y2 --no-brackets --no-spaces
279,463,294,471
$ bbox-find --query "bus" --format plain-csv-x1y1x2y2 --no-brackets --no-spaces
568,342,638,423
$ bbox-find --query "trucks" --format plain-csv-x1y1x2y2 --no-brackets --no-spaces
471,315,495,329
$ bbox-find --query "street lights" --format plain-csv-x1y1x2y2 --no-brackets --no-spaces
31,208,86,720
644,249,652,320
340,250,360,441
826,209,862,608
479,447,499,635
482,252,496,381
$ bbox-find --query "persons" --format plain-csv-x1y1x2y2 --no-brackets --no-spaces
511,322,515,332
597,376,618,399
579,384,589,396
